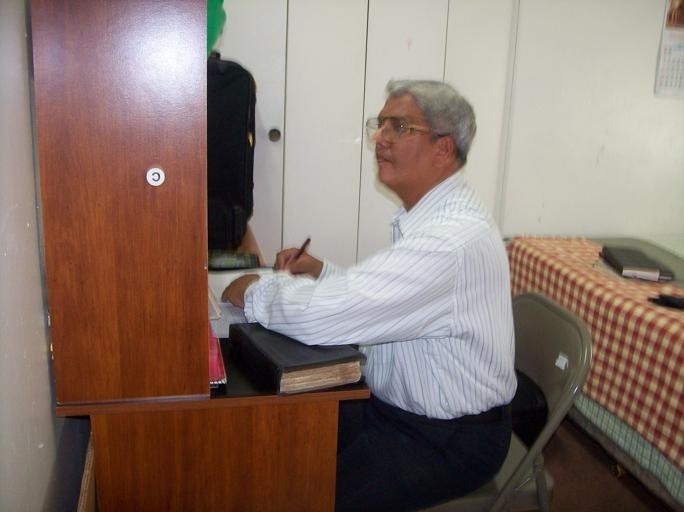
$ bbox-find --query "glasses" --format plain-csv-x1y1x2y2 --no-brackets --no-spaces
364,117,447,145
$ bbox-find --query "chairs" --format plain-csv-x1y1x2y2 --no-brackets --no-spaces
408,296,592,511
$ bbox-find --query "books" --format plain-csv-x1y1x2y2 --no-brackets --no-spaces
227,320,367,397
594,244,675,284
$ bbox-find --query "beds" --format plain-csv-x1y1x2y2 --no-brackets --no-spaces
500,236,684,510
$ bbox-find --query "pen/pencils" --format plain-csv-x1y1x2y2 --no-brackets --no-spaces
284,238,311,270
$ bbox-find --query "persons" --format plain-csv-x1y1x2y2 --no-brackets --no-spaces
218,75,523,511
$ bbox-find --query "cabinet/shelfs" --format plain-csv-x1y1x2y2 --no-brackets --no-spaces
26,0,372,512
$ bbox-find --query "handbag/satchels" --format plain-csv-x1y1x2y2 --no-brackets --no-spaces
206,47,257,249
